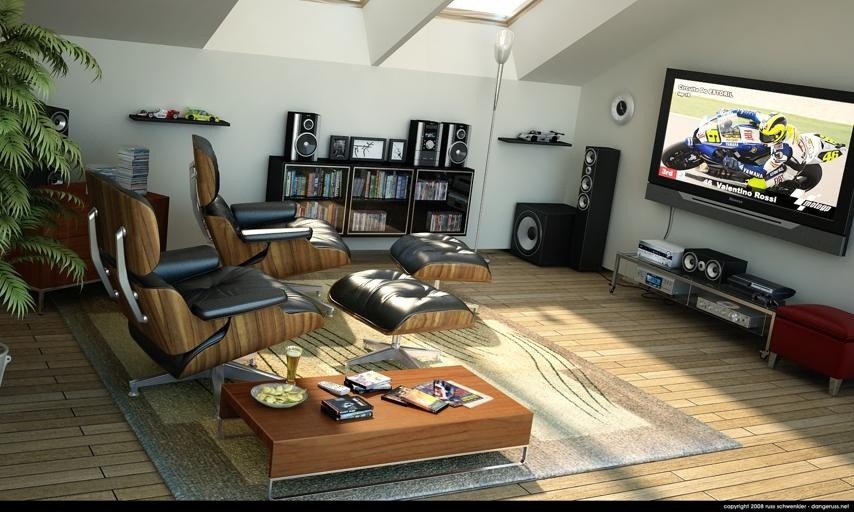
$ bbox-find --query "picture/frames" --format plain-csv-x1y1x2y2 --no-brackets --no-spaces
388,138,407,162
330,135,349,161
351,137,385,162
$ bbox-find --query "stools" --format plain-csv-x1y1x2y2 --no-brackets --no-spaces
767,303,854,397
388,230,493,311
327,267,485,373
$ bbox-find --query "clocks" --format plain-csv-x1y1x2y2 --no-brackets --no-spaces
611,91,635,124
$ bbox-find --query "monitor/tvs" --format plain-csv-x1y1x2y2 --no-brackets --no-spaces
647,68,853,237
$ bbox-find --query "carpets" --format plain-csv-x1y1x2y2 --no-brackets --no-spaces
55,279,745,501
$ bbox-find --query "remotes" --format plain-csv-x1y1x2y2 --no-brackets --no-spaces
317,380,350,396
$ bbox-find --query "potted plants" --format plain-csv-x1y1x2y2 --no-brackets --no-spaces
0,0,103,387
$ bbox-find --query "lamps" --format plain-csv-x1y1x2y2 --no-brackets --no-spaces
472,27,519,260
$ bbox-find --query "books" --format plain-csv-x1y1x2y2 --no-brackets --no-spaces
116,146,149,196
285,170,462,233
320,370,484,422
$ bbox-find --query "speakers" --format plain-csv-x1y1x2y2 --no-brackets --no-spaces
567,145,621,272
284,111,320,162
407,119,444,167
680,248,748,288
510,203,577,267
439,122,469,169
26,99,69,188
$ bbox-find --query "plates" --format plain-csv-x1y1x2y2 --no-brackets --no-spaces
250,383,308,409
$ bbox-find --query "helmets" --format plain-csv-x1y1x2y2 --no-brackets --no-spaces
758,112,787,145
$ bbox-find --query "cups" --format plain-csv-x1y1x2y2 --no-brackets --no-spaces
285,346,303,385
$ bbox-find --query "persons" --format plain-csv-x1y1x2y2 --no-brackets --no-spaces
519,129,564,143
185,108,219,122
135,107,180,120
720,108,807,190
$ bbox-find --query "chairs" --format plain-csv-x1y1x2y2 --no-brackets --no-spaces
84,170,326,416
185,132,353,279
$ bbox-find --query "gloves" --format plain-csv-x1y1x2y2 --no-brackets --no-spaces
723,155,738,170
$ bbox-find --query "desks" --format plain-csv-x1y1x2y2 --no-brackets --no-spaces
15,178,171,314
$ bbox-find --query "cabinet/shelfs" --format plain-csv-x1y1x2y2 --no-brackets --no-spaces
266,153,476,239
609,249,775,347
